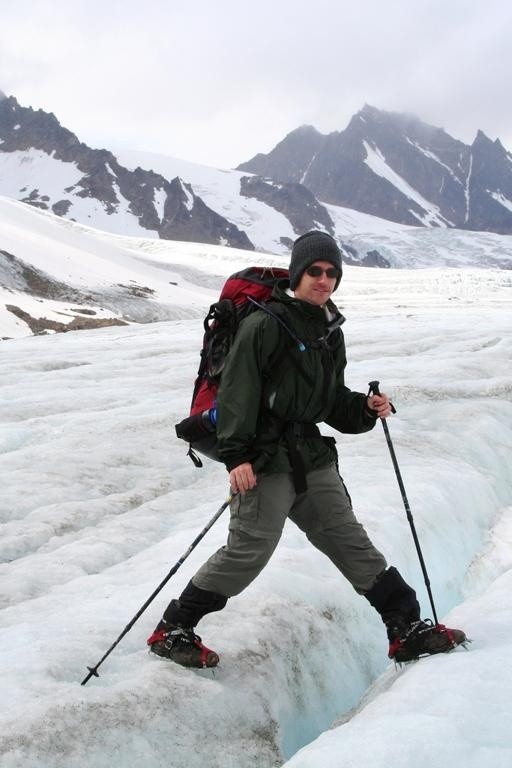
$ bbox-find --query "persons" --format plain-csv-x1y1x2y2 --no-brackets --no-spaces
142,229,473,668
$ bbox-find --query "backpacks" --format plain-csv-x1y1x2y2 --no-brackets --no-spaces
176,267,326,462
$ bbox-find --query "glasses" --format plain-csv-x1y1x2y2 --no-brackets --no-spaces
305,266,340,278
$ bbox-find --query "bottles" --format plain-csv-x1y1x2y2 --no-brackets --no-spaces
178,407,217,441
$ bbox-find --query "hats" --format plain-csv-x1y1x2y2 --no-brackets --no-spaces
289,230,342,292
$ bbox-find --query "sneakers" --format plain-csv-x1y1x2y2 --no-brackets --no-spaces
146,599,219,669
383,607,466,663
